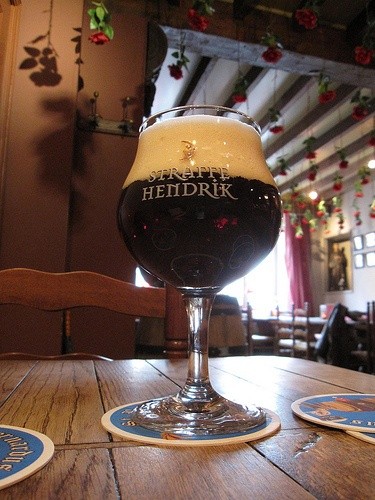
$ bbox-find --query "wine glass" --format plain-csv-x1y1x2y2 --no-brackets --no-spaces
115,105,282,435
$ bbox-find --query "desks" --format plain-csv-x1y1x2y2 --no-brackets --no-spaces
0,354,375,500
267,316,357,362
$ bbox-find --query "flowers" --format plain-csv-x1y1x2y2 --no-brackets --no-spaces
167,0,375,238
88,0,114,45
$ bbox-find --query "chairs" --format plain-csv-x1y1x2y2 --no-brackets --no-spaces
0,268,188,359
240,302,375,375
